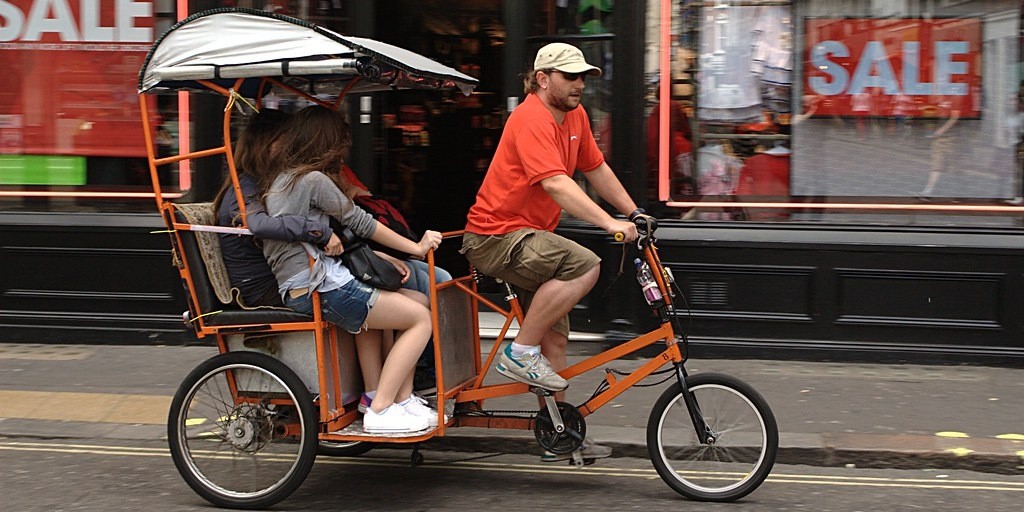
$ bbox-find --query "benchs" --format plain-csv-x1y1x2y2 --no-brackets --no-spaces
164,199,367,412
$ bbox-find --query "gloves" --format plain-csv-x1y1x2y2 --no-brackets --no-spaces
626,208,658,235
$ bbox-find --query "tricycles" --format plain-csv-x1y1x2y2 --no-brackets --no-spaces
134,7,780,512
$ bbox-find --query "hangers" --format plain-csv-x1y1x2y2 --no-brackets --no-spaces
674,131,791,168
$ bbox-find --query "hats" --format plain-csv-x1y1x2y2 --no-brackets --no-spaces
533,42,602,78
247,107,291,149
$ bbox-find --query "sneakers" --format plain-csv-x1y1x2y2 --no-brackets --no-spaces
538,435,613,461
402,394,449,427
362,402,430,433
357,391,372,413
494,342,570,392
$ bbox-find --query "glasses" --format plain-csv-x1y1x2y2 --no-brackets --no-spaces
341,133,354,140
543,69,588,81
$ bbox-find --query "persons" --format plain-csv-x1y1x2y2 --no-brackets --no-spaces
212,105,453,433
647,81,692,209
795,72,1024,222
458,42,659,462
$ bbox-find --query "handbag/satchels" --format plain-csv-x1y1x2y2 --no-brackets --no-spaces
324,223,404,292
